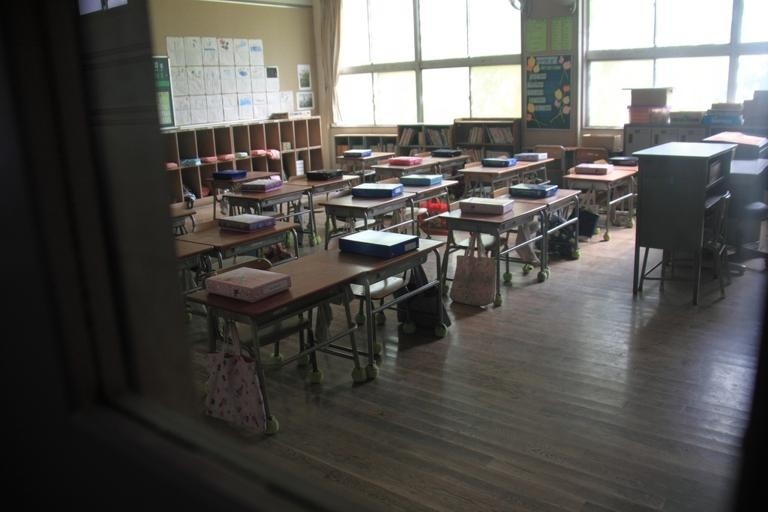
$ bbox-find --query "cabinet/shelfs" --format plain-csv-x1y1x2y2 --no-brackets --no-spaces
163,113,327,217
333,116,520,167
624,124,767,244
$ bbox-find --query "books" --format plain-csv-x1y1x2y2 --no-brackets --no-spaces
335,126,513,163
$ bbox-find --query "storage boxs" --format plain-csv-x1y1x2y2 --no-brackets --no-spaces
621,84,673,124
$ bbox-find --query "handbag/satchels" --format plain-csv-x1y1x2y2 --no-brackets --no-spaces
451,232,497,307
266,245,292,263
394,265,451,330
200,322,268,432
182,266,219,318
417,198,452,235
515,205,599,265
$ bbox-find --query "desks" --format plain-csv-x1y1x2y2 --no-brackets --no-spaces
630,136,739,307
448,152,639,306
167,151,448,436
